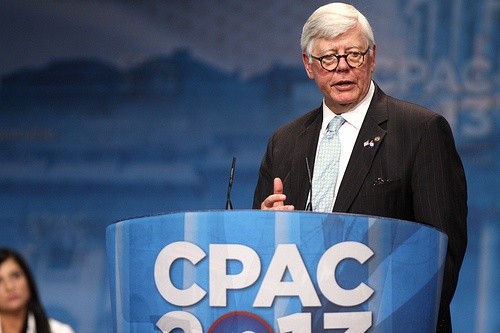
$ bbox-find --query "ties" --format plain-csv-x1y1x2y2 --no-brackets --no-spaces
312,116,347,212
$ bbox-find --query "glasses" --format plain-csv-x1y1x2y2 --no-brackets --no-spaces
310,45,372,72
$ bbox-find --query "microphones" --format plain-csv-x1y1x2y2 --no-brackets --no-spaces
304,157,312,211
226,157,236,210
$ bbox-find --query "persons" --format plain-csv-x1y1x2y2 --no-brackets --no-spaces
252,3,469,333
0,247,74,333
